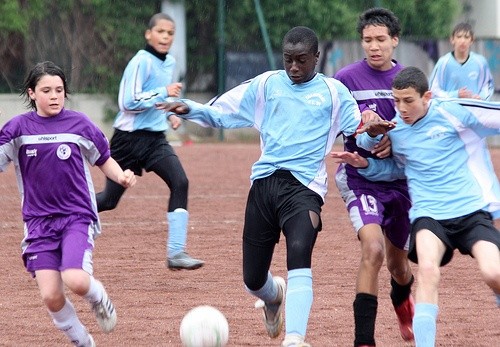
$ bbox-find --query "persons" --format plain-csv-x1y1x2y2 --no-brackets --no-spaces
95,13,206,270
330,66,500,347
156,26,397,347
0,60,136,347
429,23,495,102
333,8,415,347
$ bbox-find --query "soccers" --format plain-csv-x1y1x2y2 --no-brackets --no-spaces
179,304,229,347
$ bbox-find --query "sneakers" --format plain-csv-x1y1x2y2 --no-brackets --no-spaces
88,280,117,333
395,294,414,342
166,252,205,272
262,276,286,339
87,334,96,347
